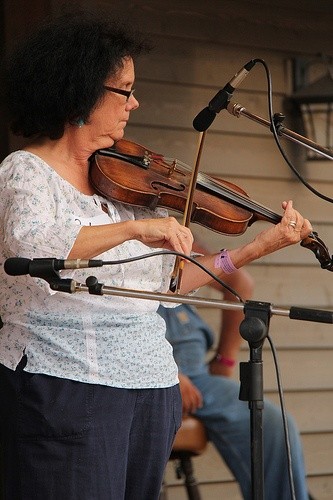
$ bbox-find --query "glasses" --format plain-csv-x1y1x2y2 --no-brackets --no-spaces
105,85,137,102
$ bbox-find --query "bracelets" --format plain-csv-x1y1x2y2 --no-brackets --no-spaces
213,349,238,368
214,248,240,276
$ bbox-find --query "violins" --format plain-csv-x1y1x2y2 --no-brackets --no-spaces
89,135,333,273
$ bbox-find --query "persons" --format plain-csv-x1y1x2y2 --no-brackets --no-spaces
157,242,310,500
0,23,313,499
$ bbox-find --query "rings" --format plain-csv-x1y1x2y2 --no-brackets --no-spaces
288,219,296,229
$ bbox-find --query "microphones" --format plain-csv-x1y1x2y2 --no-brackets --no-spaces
4,257,103,276
193,58,255,133
